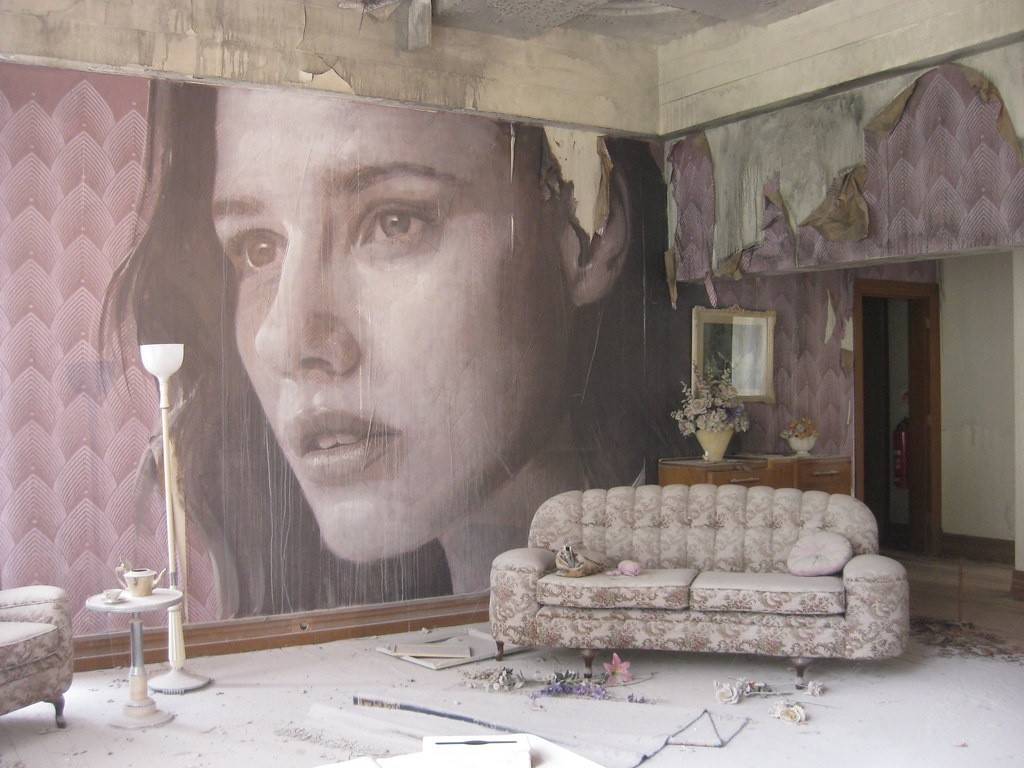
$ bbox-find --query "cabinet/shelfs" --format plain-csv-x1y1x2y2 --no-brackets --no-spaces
657,453,853,495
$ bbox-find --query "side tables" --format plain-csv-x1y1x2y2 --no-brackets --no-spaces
85,586,185,730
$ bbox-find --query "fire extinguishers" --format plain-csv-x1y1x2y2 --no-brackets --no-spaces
893,417,908,489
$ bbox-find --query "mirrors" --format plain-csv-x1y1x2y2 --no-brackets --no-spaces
691,302,777,405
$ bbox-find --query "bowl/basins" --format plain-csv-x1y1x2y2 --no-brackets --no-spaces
104,589,122,600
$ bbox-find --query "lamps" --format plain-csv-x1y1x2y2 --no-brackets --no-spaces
140,342,212,693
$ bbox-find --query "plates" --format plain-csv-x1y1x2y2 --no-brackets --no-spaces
104,599,131,603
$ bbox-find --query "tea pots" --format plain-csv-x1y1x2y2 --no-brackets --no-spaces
115,556,167,597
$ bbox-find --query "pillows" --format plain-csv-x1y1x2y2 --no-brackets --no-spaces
787,529,853,577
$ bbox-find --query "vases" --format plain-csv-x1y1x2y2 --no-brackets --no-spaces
695,430,733,462
788,437,819,457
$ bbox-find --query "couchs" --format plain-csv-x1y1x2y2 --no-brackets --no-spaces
488,483,911,691
0,585,75,728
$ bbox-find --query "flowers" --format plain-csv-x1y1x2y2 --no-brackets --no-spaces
671,358,750,438
603,649,636,687
712,676,772,707
458,666,529,691
774,692,807,725
529,669,646,705
794,680,825,697
779,416,821,440
